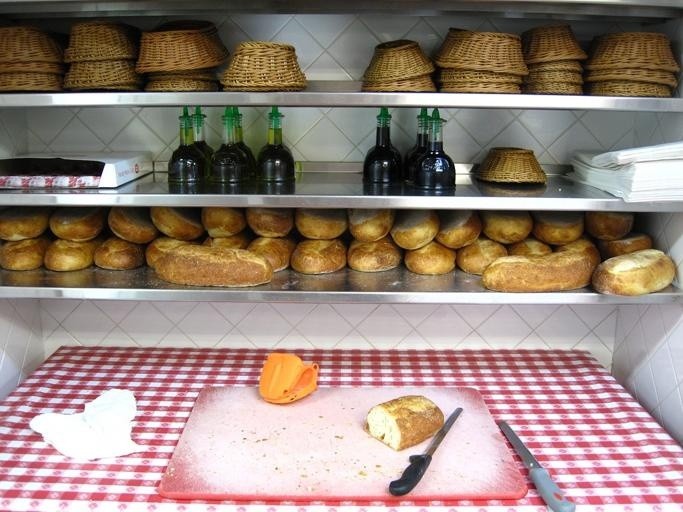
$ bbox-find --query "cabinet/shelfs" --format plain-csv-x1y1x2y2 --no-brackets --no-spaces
0,0,683,306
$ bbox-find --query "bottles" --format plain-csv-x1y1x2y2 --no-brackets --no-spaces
362,107,456,190
166,105,295,186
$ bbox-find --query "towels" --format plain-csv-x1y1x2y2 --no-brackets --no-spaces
27,389,153,463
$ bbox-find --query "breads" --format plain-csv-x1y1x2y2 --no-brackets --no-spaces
0,207,676,297
365,395,445,451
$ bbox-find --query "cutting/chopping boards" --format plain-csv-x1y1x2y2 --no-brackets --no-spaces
155,385,529,498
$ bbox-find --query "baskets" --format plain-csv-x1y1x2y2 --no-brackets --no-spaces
362,25,680,97
359,25,680,97
1,21,306,92
0,21,230,92
474,148,547,183
219,42,307,92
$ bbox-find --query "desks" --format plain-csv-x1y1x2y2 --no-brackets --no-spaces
1,347,683,510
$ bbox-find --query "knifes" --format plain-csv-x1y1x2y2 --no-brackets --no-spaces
385,404,463,497
496,419,574,512
